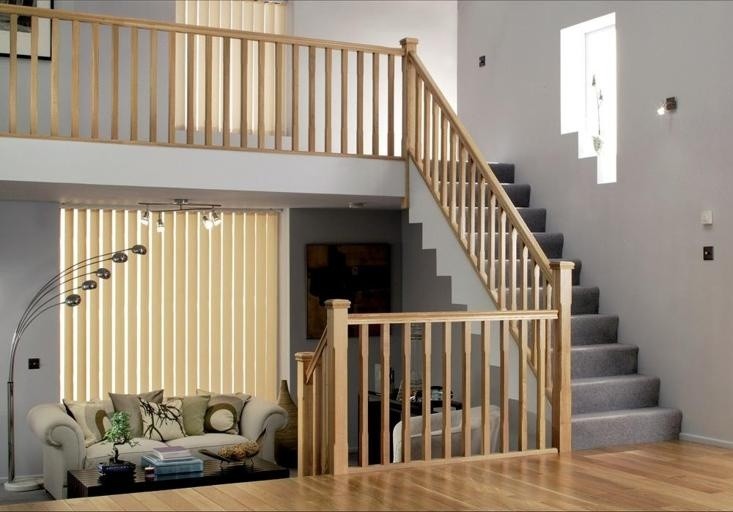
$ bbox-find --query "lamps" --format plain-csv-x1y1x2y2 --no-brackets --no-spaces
2,242,147,493
135,196,223,234
655,96,677,117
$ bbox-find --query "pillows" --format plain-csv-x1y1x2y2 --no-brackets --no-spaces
55,400,93,415
165,393,211,436
61,396,117,448
105,388,164,439
133,395,189,443
195,387,254,436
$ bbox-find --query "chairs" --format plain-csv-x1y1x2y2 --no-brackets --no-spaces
388,402,504,464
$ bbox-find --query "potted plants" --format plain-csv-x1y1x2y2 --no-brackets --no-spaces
95,409,139,482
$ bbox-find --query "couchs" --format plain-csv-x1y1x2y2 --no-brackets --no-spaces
25,392,291,502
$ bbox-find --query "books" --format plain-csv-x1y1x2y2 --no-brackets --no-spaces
148,445,193,463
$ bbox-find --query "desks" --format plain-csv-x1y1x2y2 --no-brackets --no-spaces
355,384,463,467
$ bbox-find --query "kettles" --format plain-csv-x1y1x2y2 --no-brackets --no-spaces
373,362,393,393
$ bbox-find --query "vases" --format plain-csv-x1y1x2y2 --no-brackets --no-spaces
270,377,298,473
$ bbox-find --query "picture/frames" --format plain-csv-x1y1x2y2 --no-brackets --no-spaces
0,1,53,62
303,241,394,342
